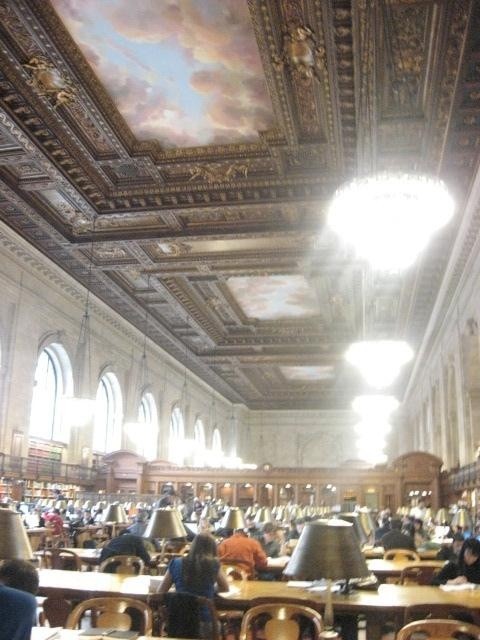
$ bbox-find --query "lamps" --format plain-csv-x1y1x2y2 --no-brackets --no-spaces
339,337,416,467
123,271,270,463
69,208,101,398
322,0,459,277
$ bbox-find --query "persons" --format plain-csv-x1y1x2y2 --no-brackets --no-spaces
1,487,480,640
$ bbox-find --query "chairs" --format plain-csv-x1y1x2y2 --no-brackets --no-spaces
0,465,480,639
